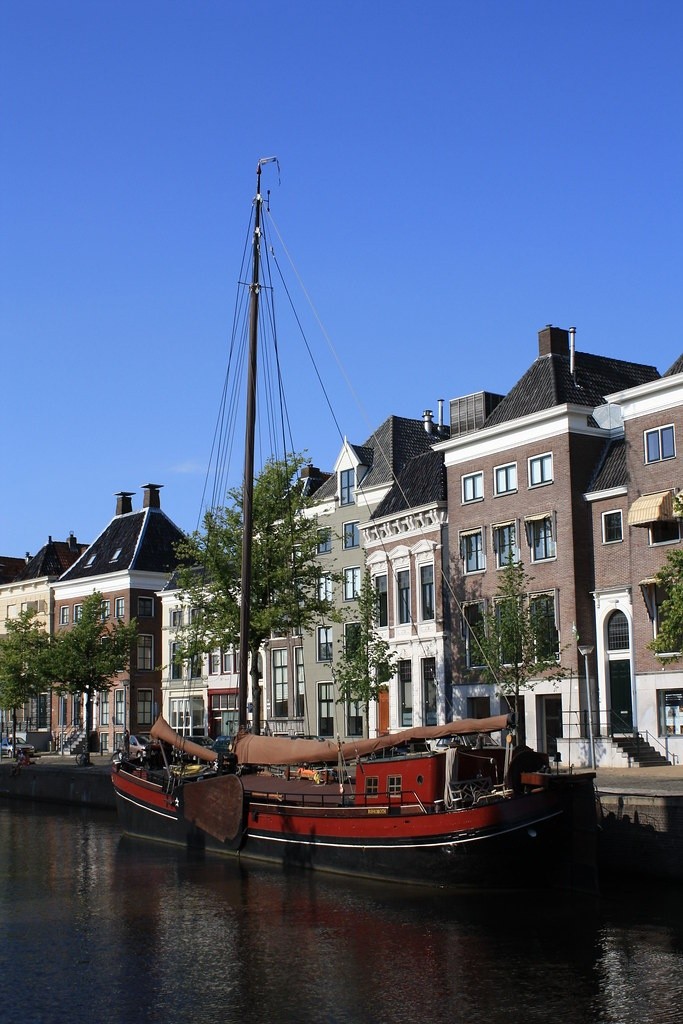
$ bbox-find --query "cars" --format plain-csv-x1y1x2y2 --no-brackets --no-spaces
2,737,35,757
431,732,508,752
117,734,153,761
184,735,215,750
212,736,231,753
278,735,324,741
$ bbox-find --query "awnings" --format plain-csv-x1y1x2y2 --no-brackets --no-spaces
628,491,676,526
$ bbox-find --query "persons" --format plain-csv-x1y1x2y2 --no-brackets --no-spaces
120,730,130,760
666,708,676,734
9,749,30,776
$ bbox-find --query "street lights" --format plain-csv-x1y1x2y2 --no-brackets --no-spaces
577,644,599,772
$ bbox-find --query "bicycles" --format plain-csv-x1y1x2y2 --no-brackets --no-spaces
75,744,90,767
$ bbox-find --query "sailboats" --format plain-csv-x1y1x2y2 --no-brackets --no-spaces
107,157,609,897
